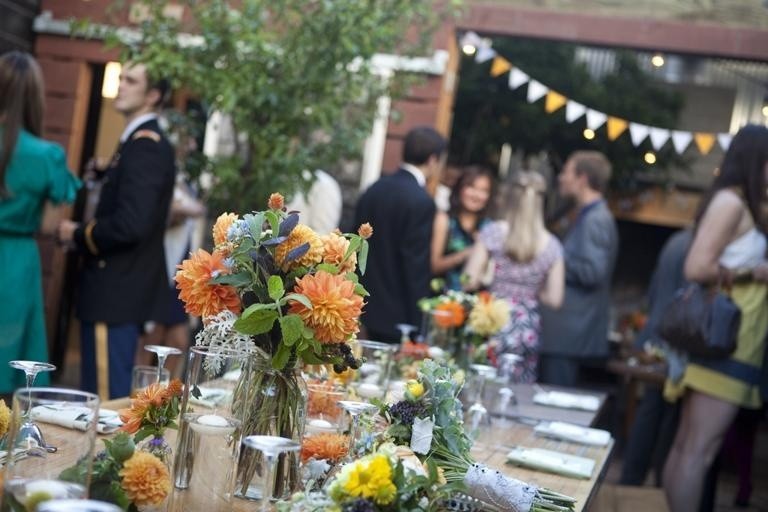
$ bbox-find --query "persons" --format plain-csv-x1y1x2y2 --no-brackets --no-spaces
460,168,567,383
430,161,492,291
658,121,768,510
0,51,82,403
352,126,448,345
616,213,768,512
537,149,618,386
135,116,345,383
57,58,177,396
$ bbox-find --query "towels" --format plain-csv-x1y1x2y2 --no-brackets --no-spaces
178,384,227,409
32,402,124,434
506,391,610,481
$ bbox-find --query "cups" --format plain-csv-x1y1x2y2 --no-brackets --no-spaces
130,364,170,413
8,386,100,512
301,310,526,455
237,434,310,511
171,344,252,488
165,413,242,511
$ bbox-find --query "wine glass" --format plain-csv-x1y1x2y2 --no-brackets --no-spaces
142,342,182,455
320,398,380,492
9,357,57,457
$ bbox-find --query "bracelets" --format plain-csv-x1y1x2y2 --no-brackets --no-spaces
735,264,753,284
451,252,461,265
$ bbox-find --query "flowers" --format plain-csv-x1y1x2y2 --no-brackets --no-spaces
114,191,509,512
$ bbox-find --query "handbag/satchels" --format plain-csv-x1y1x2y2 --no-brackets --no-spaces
654,286,741,361
